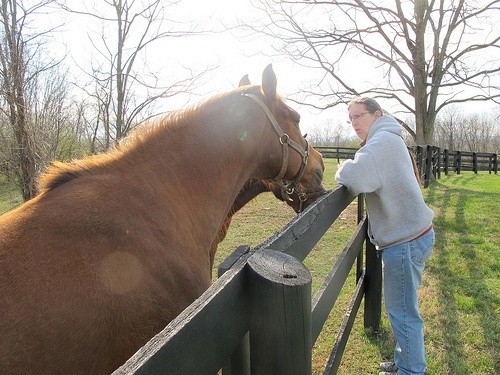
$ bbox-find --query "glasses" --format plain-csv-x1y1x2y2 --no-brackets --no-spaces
346,111,371,124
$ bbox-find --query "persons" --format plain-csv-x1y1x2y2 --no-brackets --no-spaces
334,96,438,375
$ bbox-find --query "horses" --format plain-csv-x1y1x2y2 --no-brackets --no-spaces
210,133,324,282
0,63,326,374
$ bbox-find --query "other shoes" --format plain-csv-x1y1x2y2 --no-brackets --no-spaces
379,361,398,372
375,371,398,375
